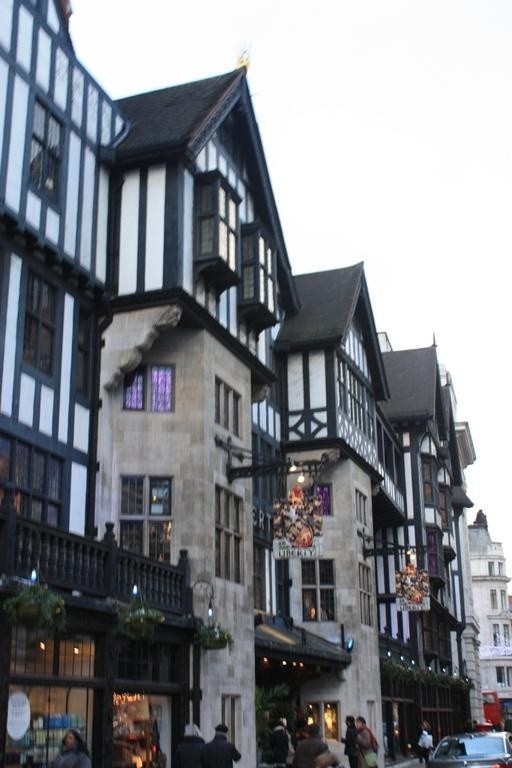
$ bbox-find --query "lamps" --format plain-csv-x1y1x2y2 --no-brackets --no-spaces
358,529,412,556
192,578,214,617
297,465,305,483
17,521,42,582
117,550,138,594
215,435,297,472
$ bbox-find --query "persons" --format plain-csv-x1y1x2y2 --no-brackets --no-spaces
50,728,92,768
269,716,379,768
171,723,205,768
417,719,433,765
201,724,242,768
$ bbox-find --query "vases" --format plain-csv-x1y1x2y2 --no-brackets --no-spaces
17,603,42,623
127,622,155,641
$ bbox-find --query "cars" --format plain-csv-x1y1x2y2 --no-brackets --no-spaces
424,730,512,767
470,723,496,731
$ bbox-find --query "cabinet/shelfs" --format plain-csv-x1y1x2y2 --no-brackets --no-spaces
19,728,83,768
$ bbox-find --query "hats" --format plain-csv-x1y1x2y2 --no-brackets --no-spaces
216,724,228,732
184,724,200,737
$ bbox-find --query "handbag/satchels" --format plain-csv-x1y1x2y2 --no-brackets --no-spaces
314,753,336,768
364,753,377,767
418,735,433,749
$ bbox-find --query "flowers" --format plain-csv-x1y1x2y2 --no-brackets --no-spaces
192,622,234,656
112,596,165,639
203,638,226,649
2,582,65,648
